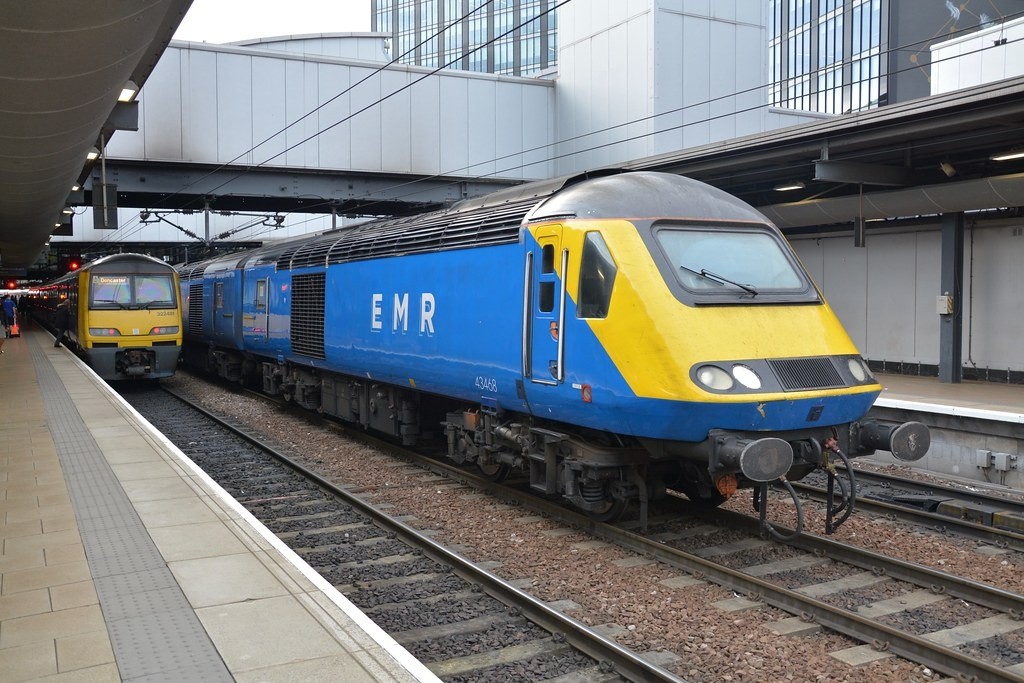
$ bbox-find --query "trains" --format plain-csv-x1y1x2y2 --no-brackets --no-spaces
172,166,932,542
22,250,184,386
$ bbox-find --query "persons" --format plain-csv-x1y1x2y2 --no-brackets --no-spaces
11,295,34,316
3,294,16,338
54,299,70,348
0,298,6,353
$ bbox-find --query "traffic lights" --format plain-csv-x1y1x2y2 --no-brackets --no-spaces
65,256,83,272
8,281,16,290
